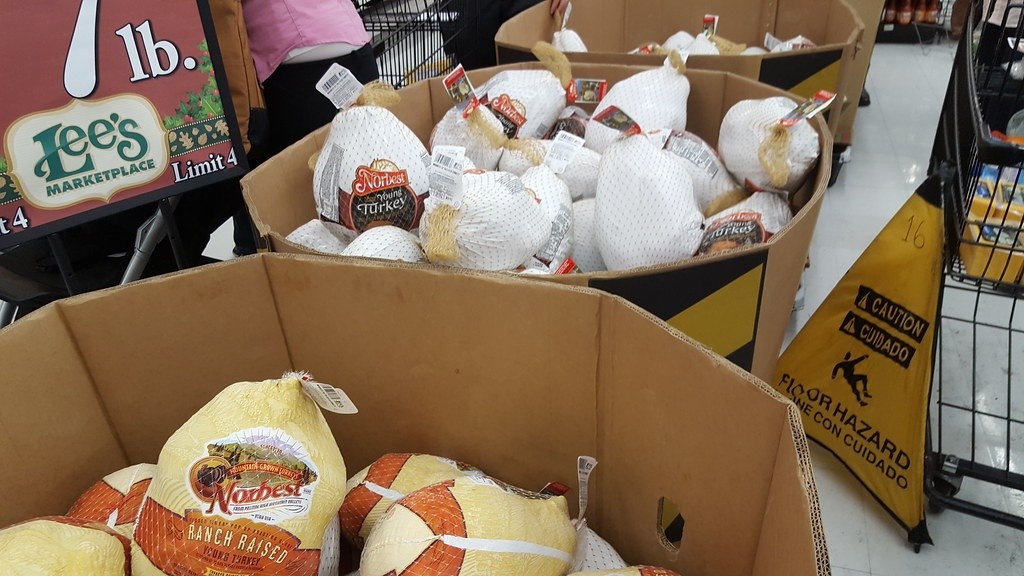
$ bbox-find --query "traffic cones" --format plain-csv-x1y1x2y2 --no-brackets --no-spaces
767,166,951,554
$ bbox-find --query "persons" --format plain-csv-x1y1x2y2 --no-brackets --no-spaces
478,0,573,64
228,1,382,255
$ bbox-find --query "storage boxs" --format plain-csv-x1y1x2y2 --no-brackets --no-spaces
2,255,835,576
491,0,864,187
243,57,835,392
